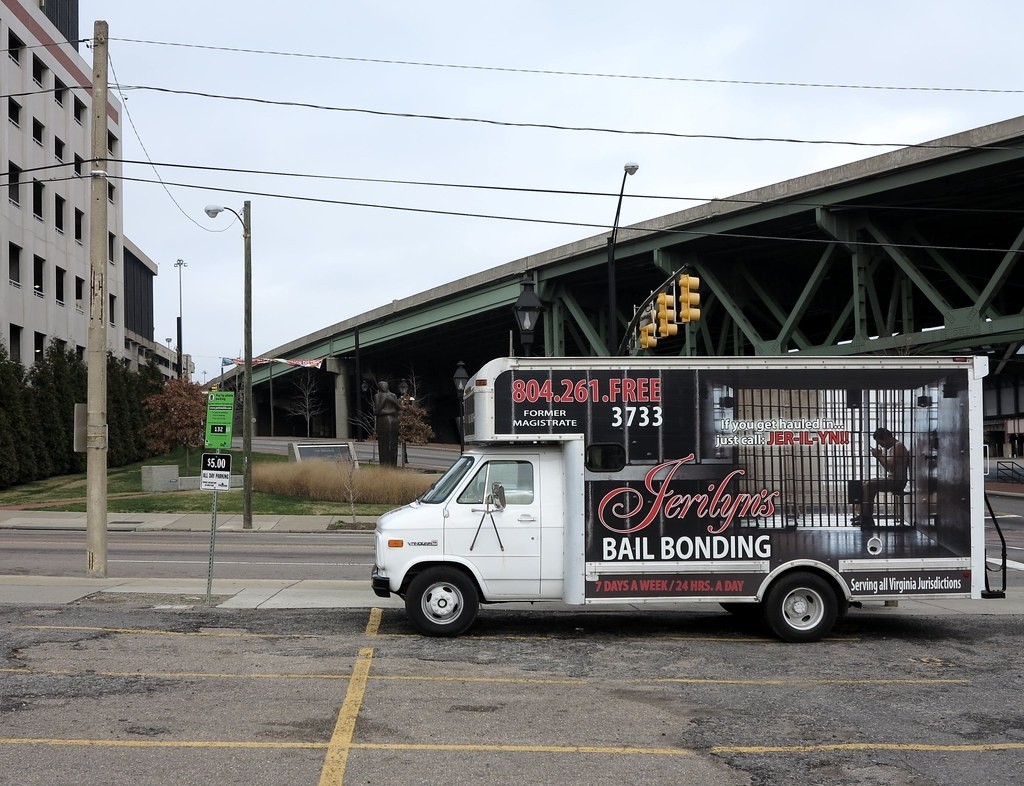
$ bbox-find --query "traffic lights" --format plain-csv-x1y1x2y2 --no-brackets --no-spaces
678,273,700,323
639,310,657,348
656,293,677,337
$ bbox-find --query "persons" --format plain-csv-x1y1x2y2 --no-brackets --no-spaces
374,382,402,467
850,428,907,525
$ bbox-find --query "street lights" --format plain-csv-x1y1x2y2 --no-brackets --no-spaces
166,338,173,381
607,162,639,357
203,370,207,384
205,201,252,529
397,378,409,464
511,277,544,357
453,360,469,456
174,258,188,379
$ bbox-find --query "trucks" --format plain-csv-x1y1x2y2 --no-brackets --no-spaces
370,357,1007,643
